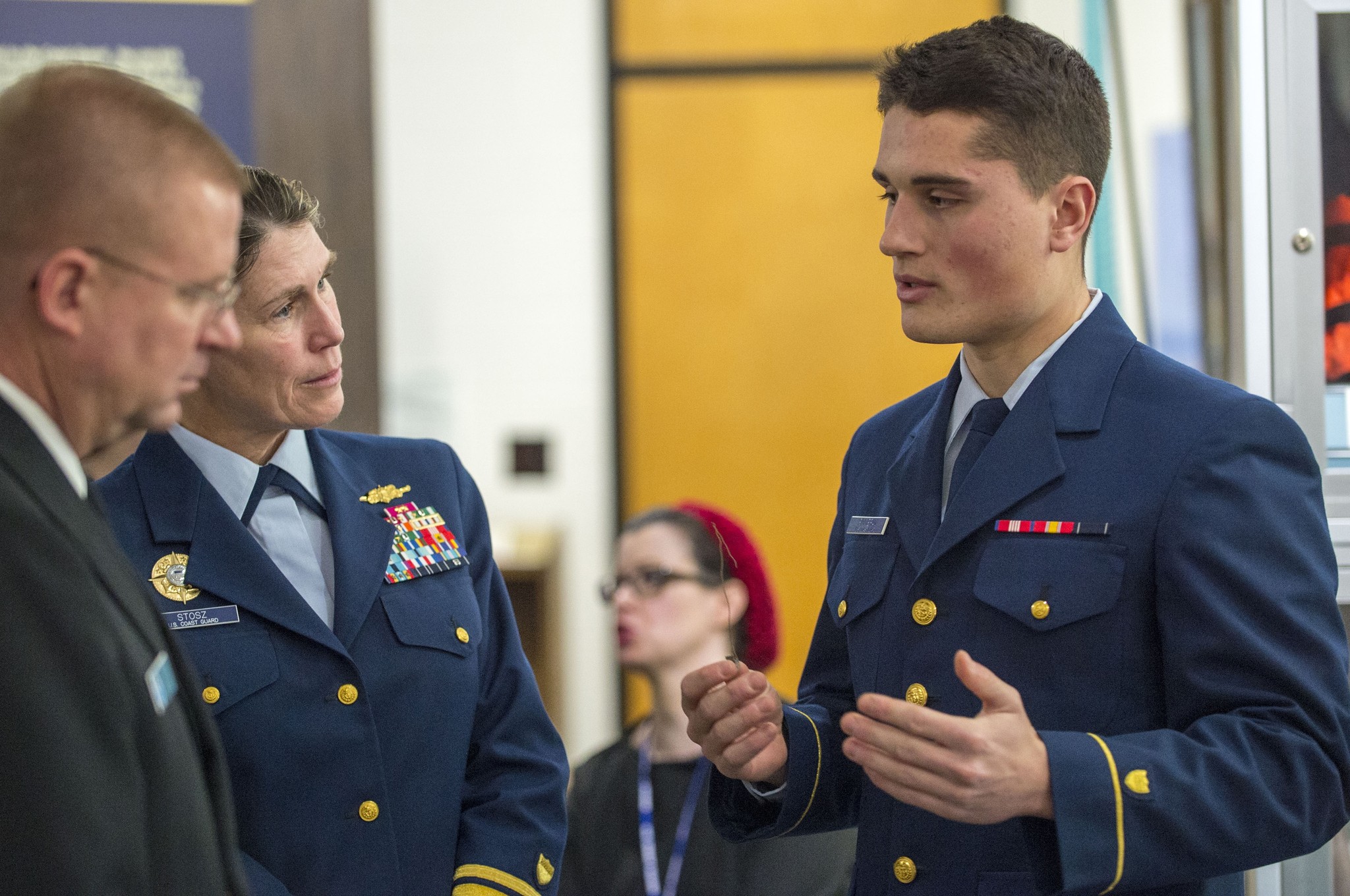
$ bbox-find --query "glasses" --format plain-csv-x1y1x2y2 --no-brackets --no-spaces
27,243,243,320
600,565,720,597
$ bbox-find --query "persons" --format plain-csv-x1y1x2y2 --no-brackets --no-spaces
681,11,1349,896
87,161,570,896
0,65,253,895
554,501,856,895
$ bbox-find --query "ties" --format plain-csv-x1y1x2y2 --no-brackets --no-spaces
945,397,1011,515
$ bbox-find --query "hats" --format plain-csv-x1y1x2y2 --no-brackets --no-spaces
680,504,777,671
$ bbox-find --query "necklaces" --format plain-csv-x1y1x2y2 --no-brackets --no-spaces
638,720,710,895
